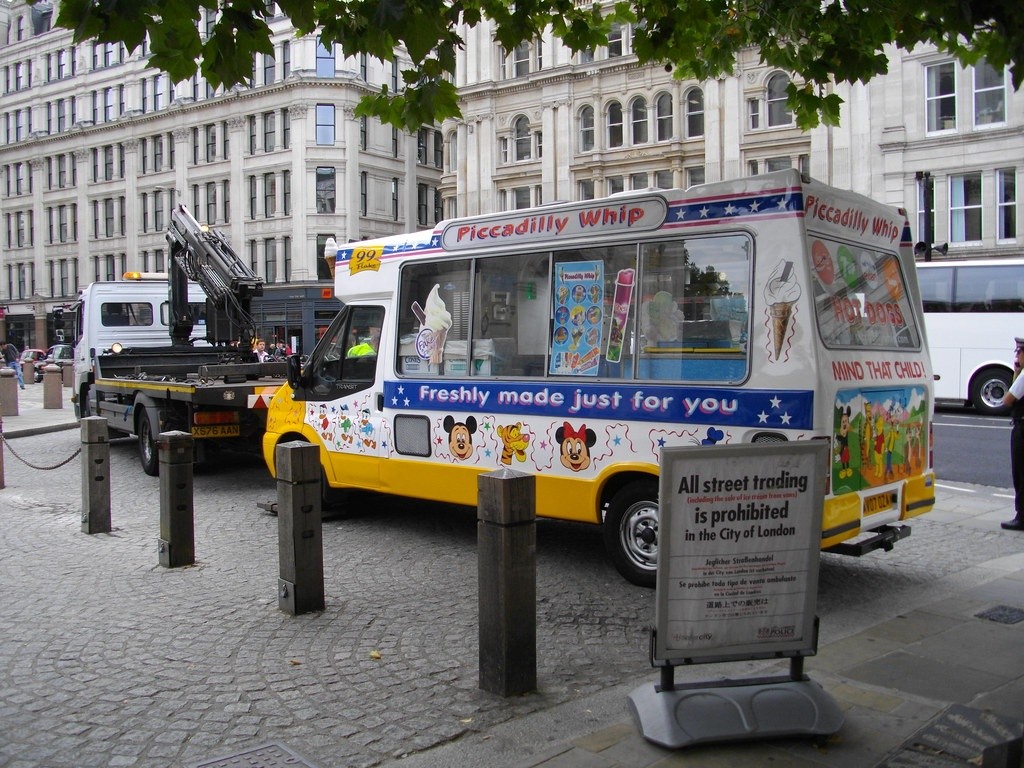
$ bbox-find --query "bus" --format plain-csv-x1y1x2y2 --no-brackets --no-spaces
913,257,1024,417
259,163,939,591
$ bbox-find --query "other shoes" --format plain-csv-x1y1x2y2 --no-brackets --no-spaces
20,386,25,389
1001,519,1024,530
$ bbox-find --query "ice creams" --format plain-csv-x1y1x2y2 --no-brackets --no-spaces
324,237,339,275
572,325,585,346
595,265,601,283
560,266,564,283
410,284,452,364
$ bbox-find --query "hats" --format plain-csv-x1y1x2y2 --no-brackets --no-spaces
1013,338,1024,351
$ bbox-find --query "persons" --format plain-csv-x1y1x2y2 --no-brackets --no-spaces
230,338,292,363
0,341,26,391
1001,338,1024,531
347,336,376,355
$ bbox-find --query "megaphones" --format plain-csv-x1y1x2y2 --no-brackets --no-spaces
934,243,948,256
914,241,928,255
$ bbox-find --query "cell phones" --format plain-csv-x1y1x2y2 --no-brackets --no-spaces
1015,362,1020,367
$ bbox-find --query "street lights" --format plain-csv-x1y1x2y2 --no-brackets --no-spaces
915,169,951,262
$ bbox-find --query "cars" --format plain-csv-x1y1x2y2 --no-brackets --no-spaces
0,343,74,382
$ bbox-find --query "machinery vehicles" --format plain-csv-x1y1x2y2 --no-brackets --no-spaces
52,202,314,478
